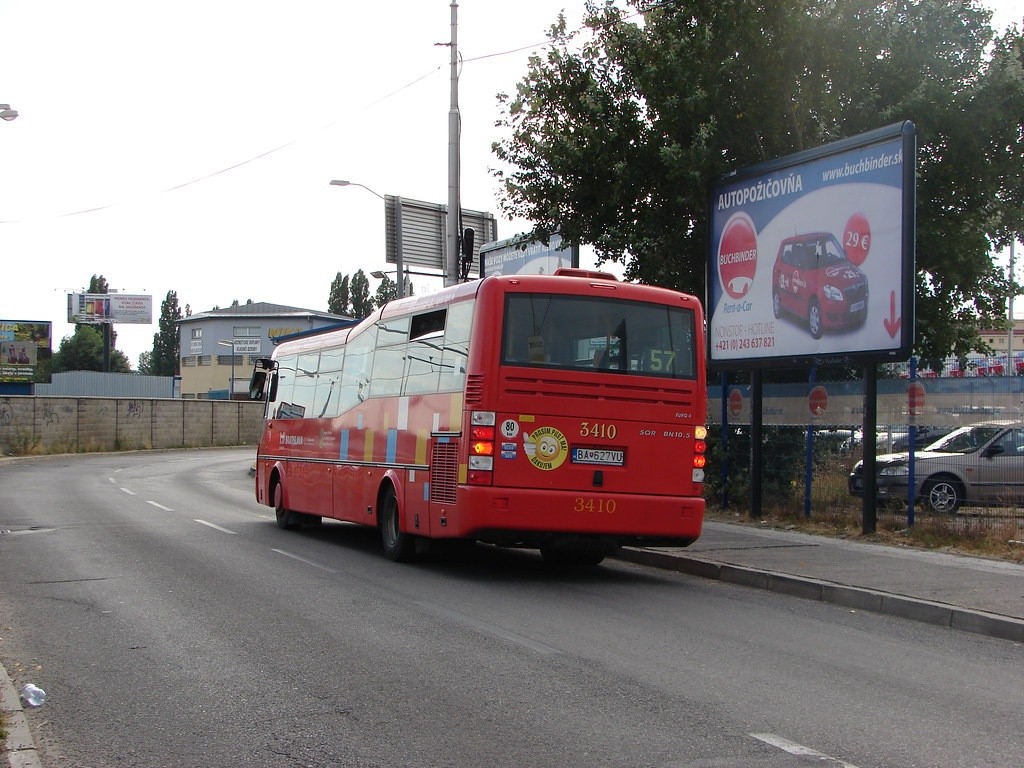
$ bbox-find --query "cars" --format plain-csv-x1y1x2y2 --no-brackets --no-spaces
847,420,1024,513
772,233,870,337
840,427,960,461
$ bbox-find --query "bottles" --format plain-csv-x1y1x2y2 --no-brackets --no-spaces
23,684,46,706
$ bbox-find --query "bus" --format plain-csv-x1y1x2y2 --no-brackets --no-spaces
247,268,713,566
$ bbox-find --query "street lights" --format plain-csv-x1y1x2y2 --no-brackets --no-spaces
369,268,410,299
217,340,234,401
330,179,403,302
